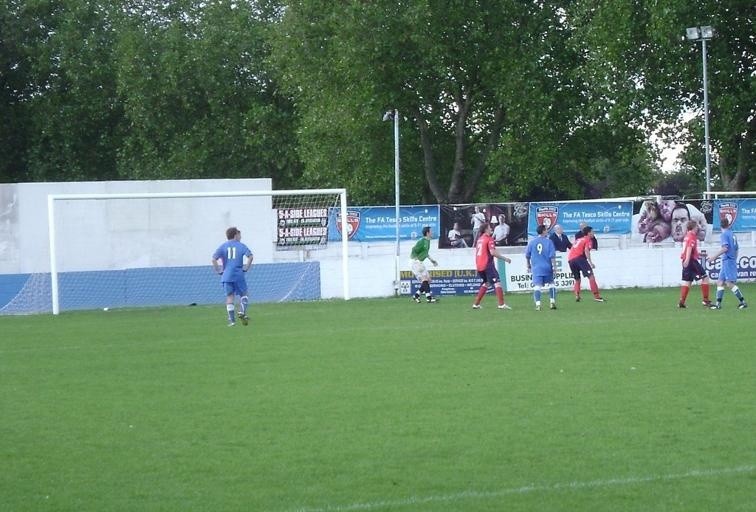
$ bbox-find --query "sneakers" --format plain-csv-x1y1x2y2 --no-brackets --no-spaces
497,304,511,310
536,306,543,312
426,297,436,303
412,295,421,303
735,303,747,310
550,304,556,310
677,301,721,311
472,304,481,309
226,320,236,327
237,312,248,326
594,298,606,302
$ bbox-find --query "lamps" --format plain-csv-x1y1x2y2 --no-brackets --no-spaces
490,215,498,232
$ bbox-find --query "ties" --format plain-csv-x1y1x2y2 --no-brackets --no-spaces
559,235,561,239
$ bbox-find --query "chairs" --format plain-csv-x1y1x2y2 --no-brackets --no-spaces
492,233,511,245
443,227,462,247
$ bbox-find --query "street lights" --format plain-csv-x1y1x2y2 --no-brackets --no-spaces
683,21,717,196
381,106,406,292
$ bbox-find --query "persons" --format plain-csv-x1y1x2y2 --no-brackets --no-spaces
470,223,513,311
470,205,487,248
211,225,254,328
447,222,469,248
676,221,716,308
525,224,559,311
567,225,608,303
491,213,511,246
635,199,708,246
575,223,598,251
549,225,572,252
707,217,749,310
408,226,440,304
